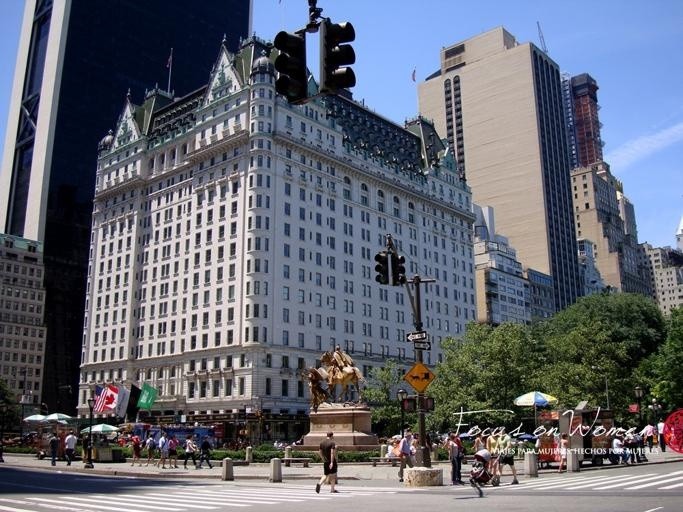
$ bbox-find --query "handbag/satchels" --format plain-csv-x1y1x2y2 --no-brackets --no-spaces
392,444,403,456
458,453,465,460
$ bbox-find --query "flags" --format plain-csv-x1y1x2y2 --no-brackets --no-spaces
92,382,158,418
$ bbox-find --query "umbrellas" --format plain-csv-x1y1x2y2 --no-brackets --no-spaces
514,389,558,429
23,412,72,439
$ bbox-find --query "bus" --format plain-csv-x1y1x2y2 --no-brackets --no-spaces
131,422,215,450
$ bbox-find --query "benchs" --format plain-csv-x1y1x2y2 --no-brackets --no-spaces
281,457,312,468
368,456,399,467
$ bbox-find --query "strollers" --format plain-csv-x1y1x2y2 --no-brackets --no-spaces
468,449,501,488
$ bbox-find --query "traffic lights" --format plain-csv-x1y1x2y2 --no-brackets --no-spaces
390,254,406,287
372,251,389,286
238,430,249,435
257,410,262,417
401,399,415,413
423,396,435,412
254,409,258,416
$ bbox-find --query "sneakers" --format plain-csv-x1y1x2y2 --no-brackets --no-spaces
316,484,321,493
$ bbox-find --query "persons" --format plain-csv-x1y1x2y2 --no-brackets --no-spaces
380,427,415,484
128,433,214,470
315,430,340,493
426,426,568,486
50,431,111,466
610,418,668,465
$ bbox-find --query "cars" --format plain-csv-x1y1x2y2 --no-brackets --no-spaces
4,430,40,448
390,432,536,445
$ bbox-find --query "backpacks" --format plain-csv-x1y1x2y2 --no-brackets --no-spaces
148,438,156,450
183,442,187,449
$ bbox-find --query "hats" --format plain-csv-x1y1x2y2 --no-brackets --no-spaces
132,436,140,442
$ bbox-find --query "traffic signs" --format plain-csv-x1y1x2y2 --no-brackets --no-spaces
404,330,428,343
412,340,432,352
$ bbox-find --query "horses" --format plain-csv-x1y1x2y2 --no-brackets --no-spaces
319,351,370,404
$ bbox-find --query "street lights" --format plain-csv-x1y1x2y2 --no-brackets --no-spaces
632,386,643,428
395,387,408,440
646,398,661,421
591,366,609,409
41,403,49,409
33,408,49,415
82,397,97,469
